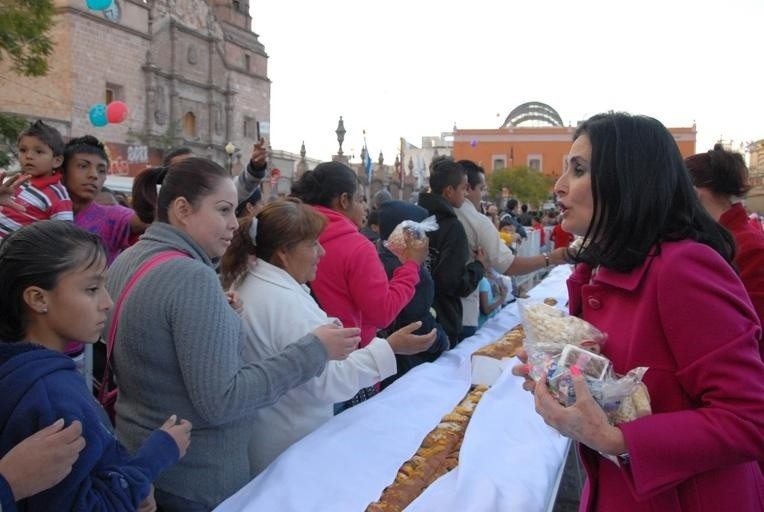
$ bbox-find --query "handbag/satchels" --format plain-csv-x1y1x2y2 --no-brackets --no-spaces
100,252,191,429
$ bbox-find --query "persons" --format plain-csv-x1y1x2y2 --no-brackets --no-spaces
289,160,430,417
0,219,194,512
477,269,509,328
684,144,764,361
457,158,578,340
95,186,118,206
499,199,527,238
162,137,269,204
518,204,532,226
511,110,764,512
0,418,87,512
218,200,438,482
361,210,380,245
0,119,74,243
416,160,488,351
486,203,498,223
0,135,150,377
375,201,451,372
113,190,129,207
531,217,546,252
549,214,575,249
236,187,265,221
497,216,523,306
100,156,362,512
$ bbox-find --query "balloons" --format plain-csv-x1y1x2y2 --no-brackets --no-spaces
469,138,477,148
105,100,128,124
86,0,112,11
88,104,108,127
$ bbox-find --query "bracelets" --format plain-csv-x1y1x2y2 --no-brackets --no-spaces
543,252,549,266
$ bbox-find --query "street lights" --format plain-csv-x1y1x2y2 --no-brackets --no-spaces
226,141,236,177
